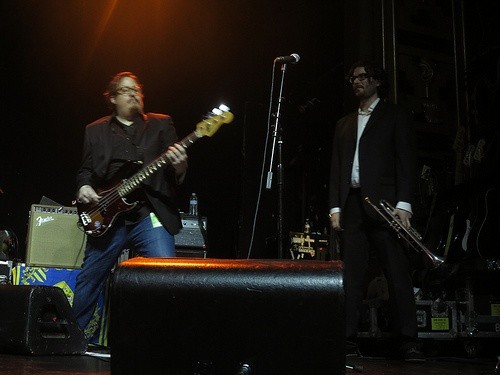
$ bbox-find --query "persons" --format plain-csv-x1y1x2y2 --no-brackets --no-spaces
72,70,188,348
327,57,428,364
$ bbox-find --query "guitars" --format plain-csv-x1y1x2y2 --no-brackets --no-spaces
77,111,235,237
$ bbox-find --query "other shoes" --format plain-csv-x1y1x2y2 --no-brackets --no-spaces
346,344,357,356
403,341,425,362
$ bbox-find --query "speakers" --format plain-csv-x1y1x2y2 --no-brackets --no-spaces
0,284,88,356
26,203,90,270
108,257,349,375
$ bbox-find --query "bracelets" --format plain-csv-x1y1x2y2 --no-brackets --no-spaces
328,212,335,218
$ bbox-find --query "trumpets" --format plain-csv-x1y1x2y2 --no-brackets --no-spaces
363,194,447,268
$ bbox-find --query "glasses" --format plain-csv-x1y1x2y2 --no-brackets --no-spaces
113,86,142,94
349,73,368,83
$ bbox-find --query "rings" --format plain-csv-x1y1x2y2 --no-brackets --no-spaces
180,150,186,154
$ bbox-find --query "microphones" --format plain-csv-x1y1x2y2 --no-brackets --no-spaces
276,53,300,64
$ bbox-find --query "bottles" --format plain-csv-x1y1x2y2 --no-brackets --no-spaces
189,193,197,216
304,219,311,233
